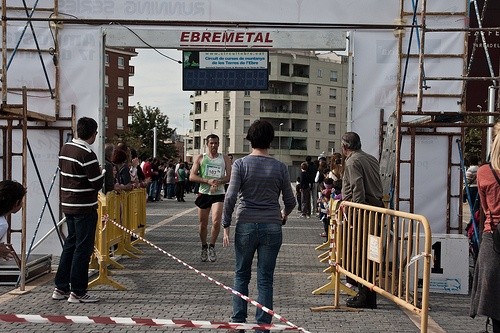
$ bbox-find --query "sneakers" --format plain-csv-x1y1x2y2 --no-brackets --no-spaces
346,295,376,307
52,288,71,299
208,243,217,262
68,291,100,303
201,243,208,261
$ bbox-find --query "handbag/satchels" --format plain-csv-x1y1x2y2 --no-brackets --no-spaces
467,208,480,239
462,189,466,202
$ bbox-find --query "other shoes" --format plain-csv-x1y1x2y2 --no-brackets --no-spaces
344,283,354,289
229,315,245,333
296,213,310,219
321,226,325,229
320,232,327,236
253,321,270,333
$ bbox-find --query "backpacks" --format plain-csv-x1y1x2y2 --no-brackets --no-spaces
490,220,500,254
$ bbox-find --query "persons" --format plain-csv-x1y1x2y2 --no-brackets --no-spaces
102,142,201,202
0,180,28,261
224,120,385,333
189,134,231,262
52,116,104,303
463,122,500,333
